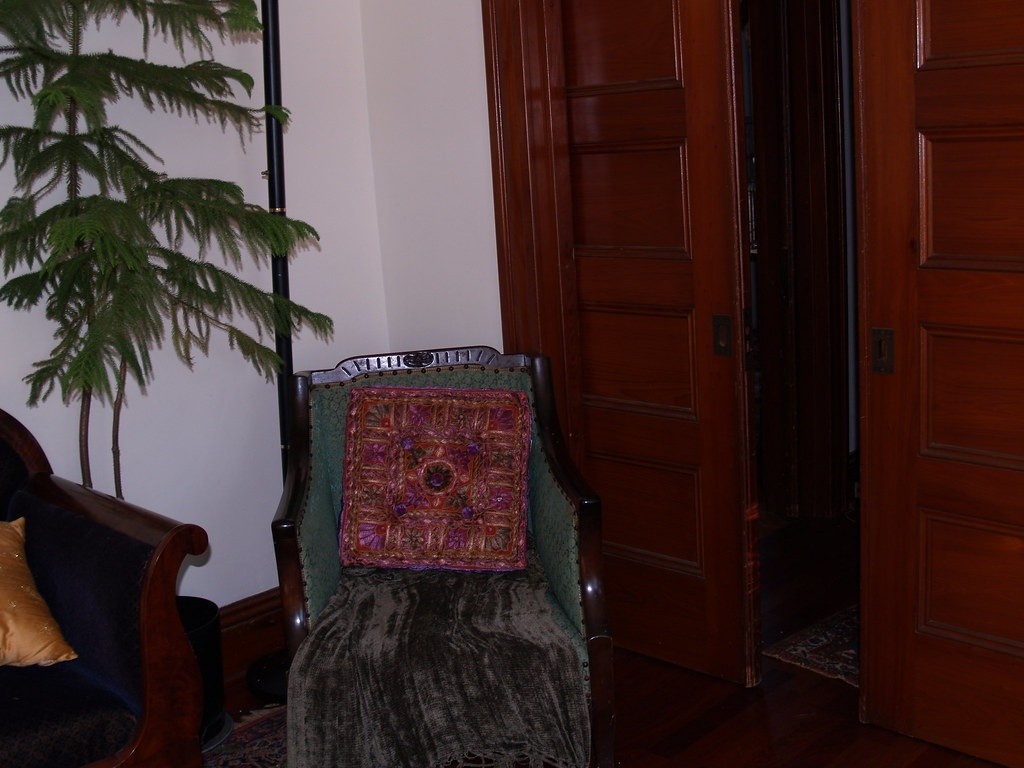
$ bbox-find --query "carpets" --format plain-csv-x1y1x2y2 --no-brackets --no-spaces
762,605,859,688
201,699,288,768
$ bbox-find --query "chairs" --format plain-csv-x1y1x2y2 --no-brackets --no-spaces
270,344,615,768
0,409,210,768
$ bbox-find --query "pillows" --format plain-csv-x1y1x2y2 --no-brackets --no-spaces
0,516,78,669
339,387,531,572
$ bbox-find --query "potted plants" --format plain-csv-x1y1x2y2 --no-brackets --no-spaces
0,0,335,749
